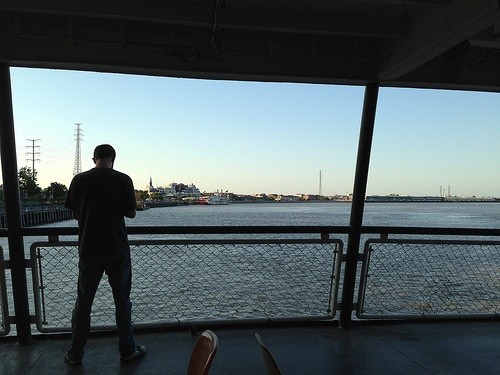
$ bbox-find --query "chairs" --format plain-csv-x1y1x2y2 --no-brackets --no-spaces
187,330,218,375
254,333,282,375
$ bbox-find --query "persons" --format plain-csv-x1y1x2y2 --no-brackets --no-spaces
62,144,148,367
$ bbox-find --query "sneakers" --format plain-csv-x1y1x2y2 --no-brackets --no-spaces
120,345,147,361
65,349,82,364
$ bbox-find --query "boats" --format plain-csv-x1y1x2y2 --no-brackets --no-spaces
136,188,230,211
